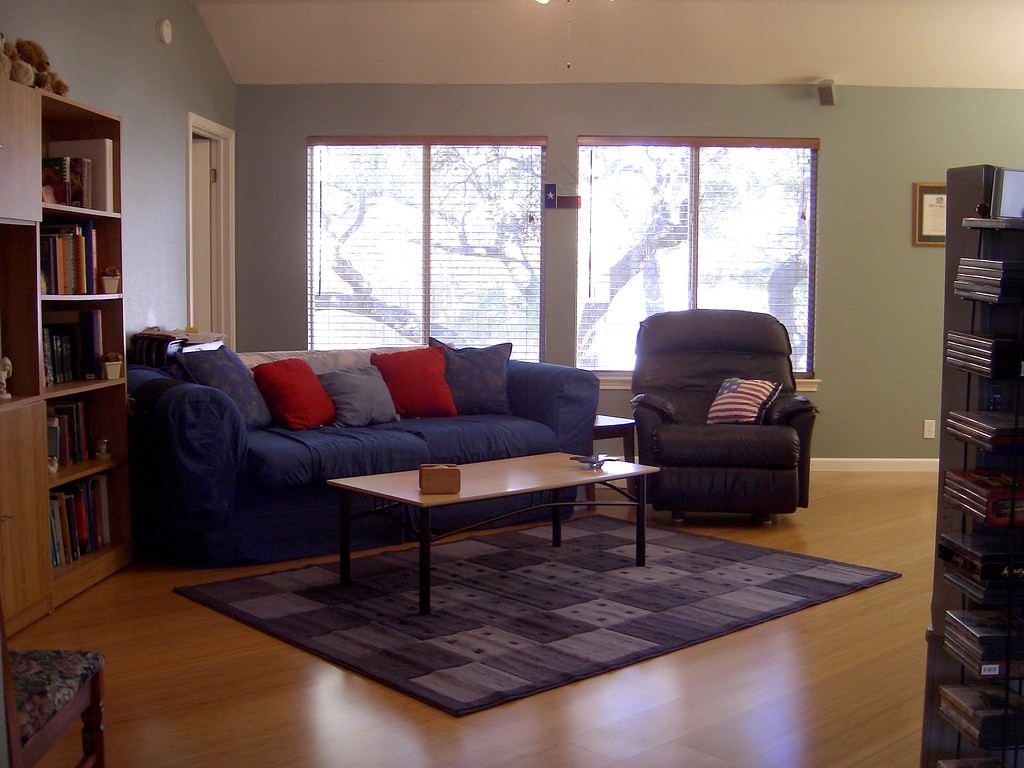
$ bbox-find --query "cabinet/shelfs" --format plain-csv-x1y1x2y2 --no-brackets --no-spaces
0,77,134,640
919,163,1024,768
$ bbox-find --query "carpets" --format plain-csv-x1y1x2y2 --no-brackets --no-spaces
171,513,903,718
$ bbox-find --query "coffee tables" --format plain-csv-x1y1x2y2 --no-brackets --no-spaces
328,452,662,616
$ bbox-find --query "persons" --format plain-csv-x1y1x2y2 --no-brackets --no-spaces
0,356,12,394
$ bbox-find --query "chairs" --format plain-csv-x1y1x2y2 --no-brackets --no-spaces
0,611,105,768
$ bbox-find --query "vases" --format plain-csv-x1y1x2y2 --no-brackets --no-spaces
102,361,121,380
101,276,120,294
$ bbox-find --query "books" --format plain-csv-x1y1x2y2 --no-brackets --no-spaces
946,329,1024,378
945,409,1024,454
40,219,98,295
178,340,221,353
49,474,111,567
944,468,1024,525
42,309,104,387
42,138,113,212
954,257,1024,304
47,400,88,466
943,608,1024,678
937,681,1024,768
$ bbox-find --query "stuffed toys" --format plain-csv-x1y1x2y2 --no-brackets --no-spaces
0,31,70,97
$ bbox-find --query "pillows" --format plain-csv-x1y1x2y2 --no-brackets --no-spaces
429,336,513,417
705,377,784,425
131,335,271,427
250,358,336,431
370,345,457,419
316,365,401,427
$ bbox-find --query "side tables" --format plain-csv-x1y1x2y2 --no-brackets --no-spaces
587,414,636,511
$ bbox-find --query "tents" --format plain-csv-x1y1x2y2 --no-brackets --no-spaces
940,531,1024,606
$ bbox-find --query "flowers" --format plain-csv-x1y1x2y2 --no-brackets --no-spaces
100,265,119,277
101,351,123,362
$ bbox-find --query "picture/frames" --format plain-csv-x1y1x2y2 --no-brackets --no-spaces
912,181,946,249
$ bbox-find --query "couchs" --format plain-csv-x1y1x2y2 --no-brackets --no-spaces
627,308,820,524
126,348,600,568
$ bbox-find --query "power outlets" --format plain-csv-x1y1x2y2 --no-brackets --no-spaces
923,420,936,439
